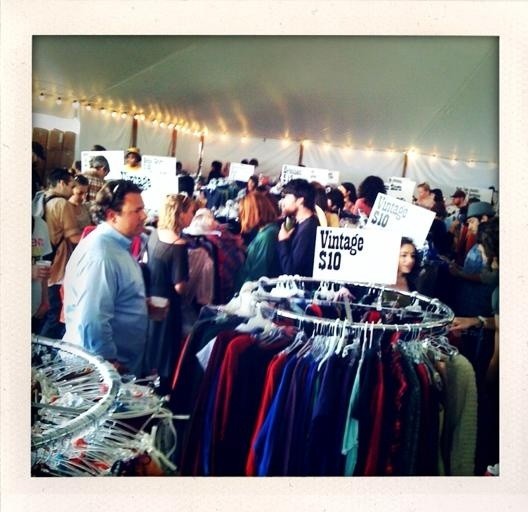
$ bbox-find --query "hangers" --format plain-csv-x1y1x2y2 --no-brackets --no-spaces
194,274,478,391
31,336,191,478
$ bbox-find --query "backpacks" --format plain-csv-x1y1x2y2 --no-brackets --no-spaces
32,191,64,220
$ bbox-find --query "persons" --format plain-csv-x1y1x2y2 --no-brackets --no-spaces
32,138,499,476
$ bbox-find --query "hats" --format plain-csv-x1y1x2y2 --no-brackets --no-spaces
452,191,465,197
466,202,495,218
